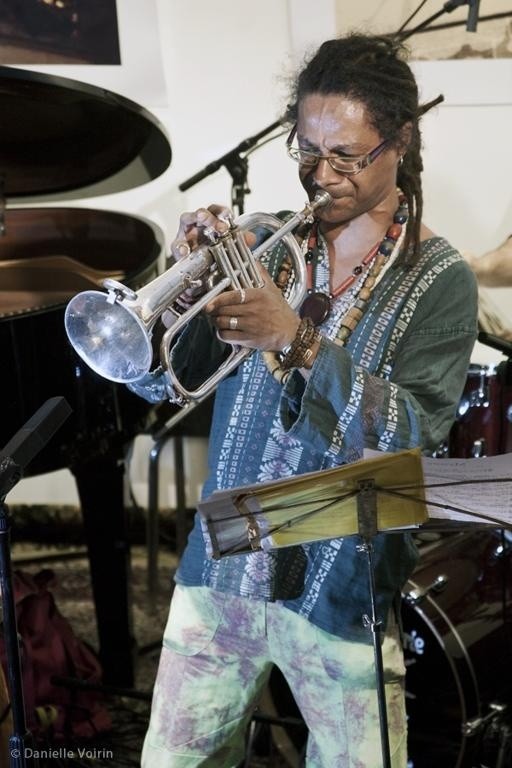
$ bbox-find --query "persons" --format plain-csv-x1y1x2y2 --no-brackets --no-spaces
124,31,480,768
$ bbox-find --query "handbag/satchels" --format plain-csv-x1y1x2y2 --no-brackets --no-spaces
15,587,118,745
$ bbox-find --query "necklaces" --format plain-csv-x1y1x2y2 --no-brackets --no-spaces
260,185,408,385
299,220,380,329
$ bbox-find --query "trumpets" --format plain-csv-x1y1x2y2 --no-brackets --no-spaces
65,191,330,439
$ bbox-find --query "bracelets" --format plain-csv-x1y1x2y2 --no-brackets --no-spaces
280,317,323,371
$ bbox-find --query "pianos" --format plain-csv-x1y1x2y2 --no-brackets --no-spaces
0,66,170,767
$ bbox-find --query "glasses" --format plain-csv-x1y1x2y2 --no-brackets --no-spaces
285,119,400,175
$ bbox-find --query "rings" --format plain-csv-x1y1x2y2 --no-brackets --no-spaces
239,289,246,304
230,317,238,330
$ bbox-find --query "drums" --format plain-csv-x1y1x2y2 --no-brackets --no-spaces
435,330,511,458
258,518,512,768
